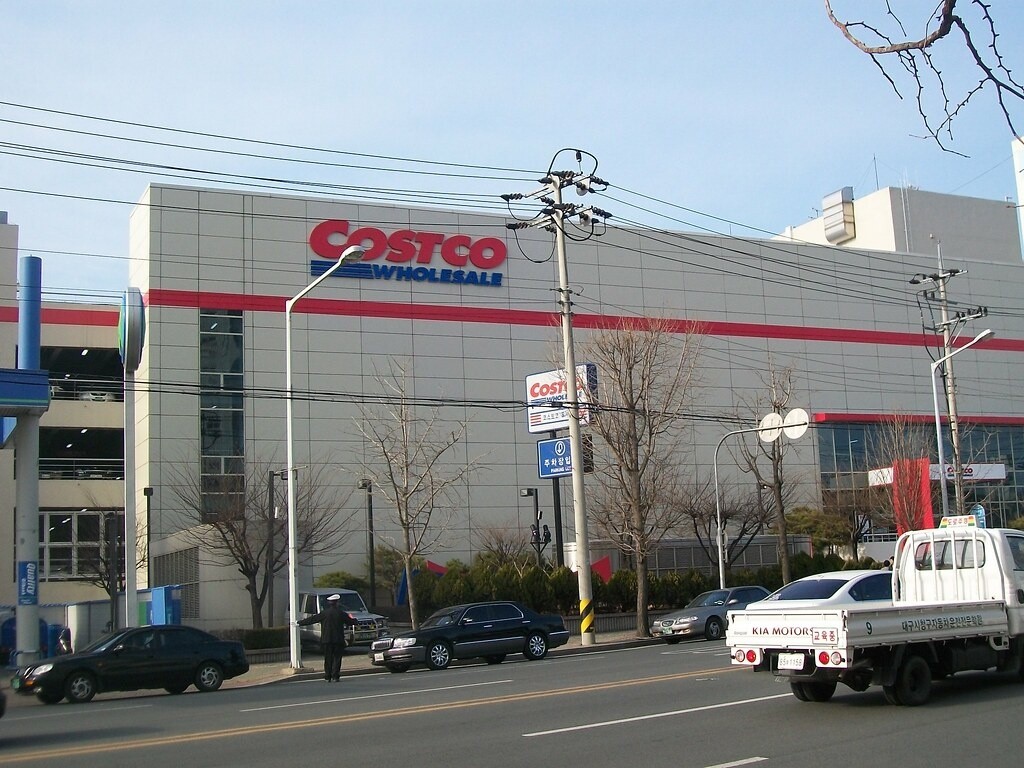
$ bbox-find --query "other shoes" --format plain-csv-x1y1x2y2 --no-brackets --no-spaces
325,679,331,682
333,679,340,682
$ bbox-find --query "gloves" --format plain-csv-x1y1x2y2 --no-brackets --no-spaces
291,621,299,628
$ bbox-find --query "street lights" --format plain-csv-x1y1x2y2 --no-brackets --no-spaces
929,329,997,517
277,243,369,676
356,478,378,612
520,488,541,568
142,484,153,591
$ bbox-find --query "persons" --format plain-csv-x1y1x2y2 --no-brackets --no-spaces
881,556,894,571
295,594,358,683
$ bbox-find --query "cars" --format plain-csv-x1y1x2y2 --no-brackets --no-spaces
367,601,571,673
10,623,250,705
747,570,902,609
650,586,772,644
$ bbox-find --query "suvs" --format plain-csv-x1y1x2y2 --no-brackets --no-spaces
283,587,391,654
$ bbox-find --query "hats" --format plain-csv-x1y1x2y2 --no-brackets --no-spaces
327,594,341,605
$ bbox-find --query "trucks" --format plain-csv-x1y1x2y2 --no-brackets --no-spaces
727,525,1024,705
